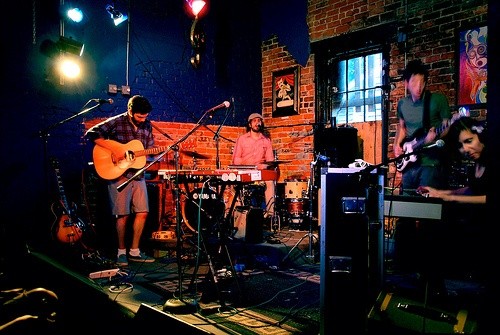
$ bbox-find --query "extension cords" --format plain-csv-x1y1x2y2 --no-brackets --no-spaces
90,269,117,279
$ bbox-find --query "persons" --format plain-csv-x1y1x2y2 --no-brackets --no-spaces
415,117,487,294
84,95,166,266
394,58,452,196
231,112,277,217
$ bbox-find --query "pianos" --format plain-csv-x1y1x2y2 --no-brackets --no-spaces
163,169,279,269
383,194,448,328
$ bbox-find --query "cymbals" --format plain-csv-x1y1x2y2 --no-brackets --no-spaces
182,150,209,159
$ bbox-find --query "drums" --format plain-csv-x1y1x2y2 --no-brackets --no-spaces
181,187,225,233
284,178,308,200
288,200,304,215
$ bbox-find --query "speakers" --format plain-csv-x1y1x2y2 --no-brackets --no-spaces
314,126,357,185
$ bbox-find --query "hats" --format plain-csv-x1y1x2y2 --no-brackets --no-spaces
248,113,263,122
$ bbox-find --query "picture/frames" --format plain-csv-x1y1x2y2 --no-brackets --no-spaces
272,66,300,118
455,21,487,110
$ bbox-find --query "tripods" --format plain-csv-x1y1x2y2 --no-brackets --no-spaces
280,155,327,265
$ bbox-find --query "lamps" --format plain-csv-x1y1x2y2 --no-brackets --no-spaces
106,5,128,26
57,37,84,57
40,39,60,60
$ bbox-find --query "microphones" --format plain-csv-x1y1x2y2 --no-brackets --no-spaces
257,122,263,131
91,98,114,104
206,101,231,114
381,83,397,91
417,140,445,152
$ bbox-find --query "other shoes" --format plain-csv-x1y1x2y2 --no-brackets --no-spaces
268,238,281,244
128,252,155,263
115,254,128,266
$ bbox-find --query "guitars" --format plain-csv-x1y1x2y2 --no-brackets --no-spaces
50,159,84,244
393,106,470,172
93,139,181,180
